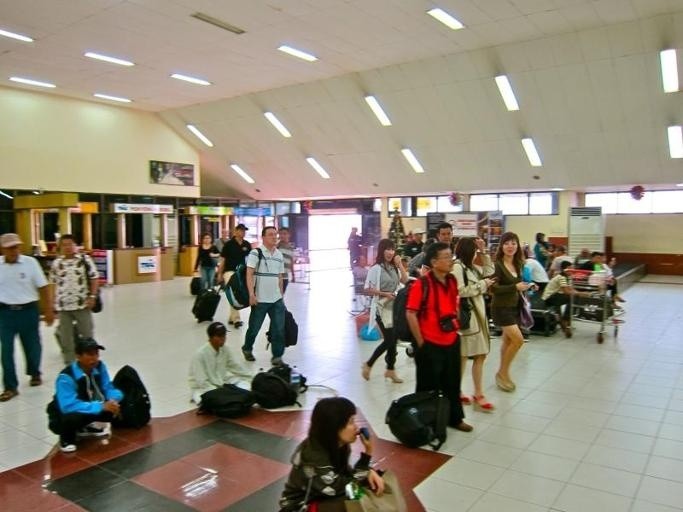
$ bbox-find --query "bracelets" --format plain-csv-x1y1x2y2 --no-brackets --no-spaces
89,294,97,299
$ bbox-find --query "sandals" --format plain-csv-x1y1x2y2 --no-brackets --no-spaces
28,373,43,387
460,389,496,413
0,388,19,402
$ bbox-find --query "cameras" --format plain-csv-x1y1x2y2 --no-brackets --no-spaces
528,283,535,289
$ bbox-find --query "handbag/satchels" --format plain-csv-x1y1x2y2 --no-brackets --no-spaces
578,291,614,321
87,288,103,314
356,307,382,342
267,310,300,349
520,297,535,331
459,297,472,332
308,467,408,512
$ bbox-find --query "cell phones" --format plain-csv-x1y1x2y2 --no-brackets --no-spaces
360,427,369,442
491,276,500,282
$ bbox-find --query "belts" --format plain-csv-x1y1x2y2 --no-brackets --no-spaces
0,302,41,313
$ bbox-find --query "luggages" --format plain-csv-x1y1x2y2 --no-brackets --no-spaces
189,269,205,297
193,287,222,322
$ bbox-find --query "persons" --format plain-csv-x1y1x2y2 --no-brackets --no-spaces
187,223,296,405
278,397,386,512
49,234,100,365
46,337,124,452
0,234,55,401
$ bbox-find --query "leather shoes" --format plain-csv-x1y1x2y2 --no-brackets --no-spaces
494,373,516,393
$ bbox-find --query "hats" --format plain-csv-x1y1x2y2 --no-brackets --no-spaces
411,226,427,236
206,322,230,337
74,337,105,353
0,232,24,250
236,222,249,231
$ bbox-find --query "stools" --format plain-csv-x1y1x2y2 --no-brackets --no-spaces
523,303,562,338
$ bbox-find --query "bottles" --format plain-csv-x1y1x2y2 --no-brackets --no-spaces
289,365,300,392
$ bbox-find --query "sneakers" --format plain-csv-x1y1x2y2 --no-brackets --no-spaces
270,357,286,369
240,344,255,362
448,418,474,432
58,435,79,454
76,423,111,438
227,319,245,329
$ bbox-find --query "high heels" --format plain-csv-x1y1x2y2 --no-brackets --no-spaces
362,362,373,382
384,370,405,385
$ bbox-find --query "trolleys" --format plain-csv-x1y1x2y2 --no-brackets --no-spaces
352,257,376,302
564,268,621,342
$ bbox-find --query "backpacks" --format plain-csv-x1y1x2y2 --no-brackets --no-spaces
385,388,452,448
224,247,263,311
392,278,433,345
109,365,152,430
252,365,307,409
197,382,257,419
